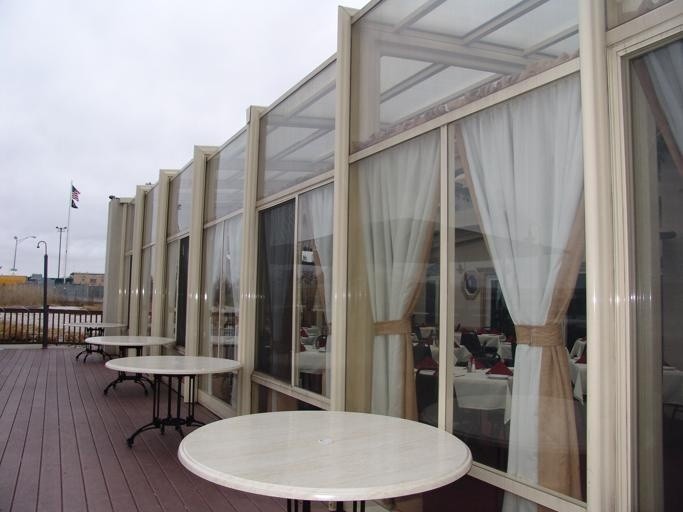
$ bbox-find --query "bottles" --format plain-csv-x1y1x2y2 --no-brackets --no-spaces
466,356,475,373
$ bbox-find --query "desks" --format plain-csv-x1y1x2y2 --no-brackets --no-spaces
104,355,244,451
85,335,179,399
175,409,476,511
61,319,131,366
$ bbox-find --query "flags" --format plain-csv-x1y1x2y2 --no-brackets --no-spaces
71,185,81,200
70,200,79,209
